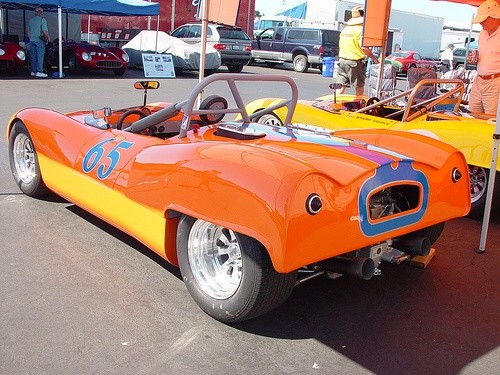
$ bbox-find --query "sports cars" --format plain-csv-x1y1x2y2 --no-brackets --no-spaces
43,37,130,77
0,40,26,77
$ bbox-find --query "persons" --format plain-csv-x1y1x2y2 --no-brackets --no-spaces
332,6,380,96
468,0,500,116
26,8,51,77
441,43,455,72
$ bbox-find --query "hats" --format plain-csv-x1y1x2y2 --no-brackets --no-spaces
35,7,43,12
472,0,500,24
346,6,365,25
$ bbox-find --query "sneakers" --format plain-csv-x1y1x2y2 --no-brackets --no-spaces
31,72,35,77
36,72,48,77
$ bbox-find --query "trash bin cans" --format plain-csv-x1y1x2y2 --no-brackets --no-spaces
321,56,337,77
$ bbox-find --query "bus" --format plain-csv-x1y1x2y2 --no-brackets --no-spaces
257,15,296,34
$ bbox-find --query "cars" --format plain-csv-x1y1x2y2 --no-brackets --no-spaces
6,72,473,326
234,78,500,221
386,51,438,76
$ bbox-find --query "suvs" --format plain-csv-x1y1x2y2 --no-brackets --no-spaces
170,22,252,74
451,47,478,71
245,26,341,73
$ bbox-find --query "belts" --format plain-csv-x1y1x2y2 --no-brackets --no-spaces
478,74,500,80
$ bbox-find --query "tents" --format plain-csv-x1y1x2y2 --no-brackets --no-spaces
430,0,500,78
0,0,160,78
